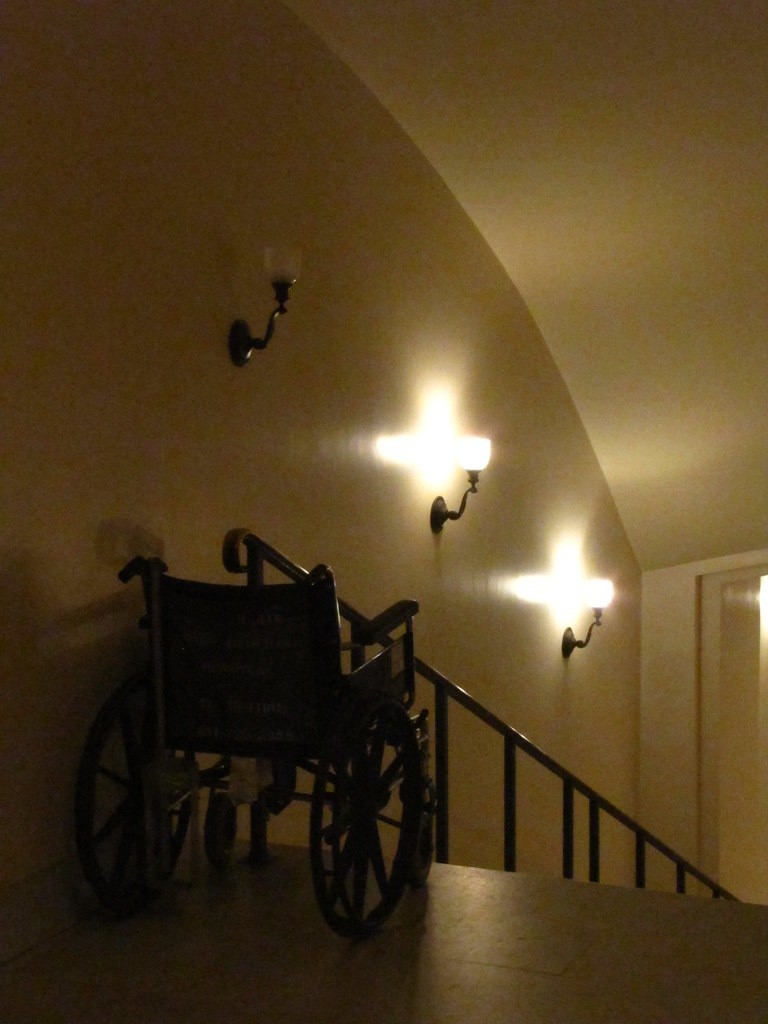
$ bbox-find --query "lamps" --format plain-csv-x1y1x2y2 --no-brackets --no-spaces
227,244,301,368
430,436,491,533
562,577,614,659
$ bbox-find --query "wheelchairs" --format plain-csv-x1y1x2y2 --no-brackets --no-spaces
72,549,437,938
